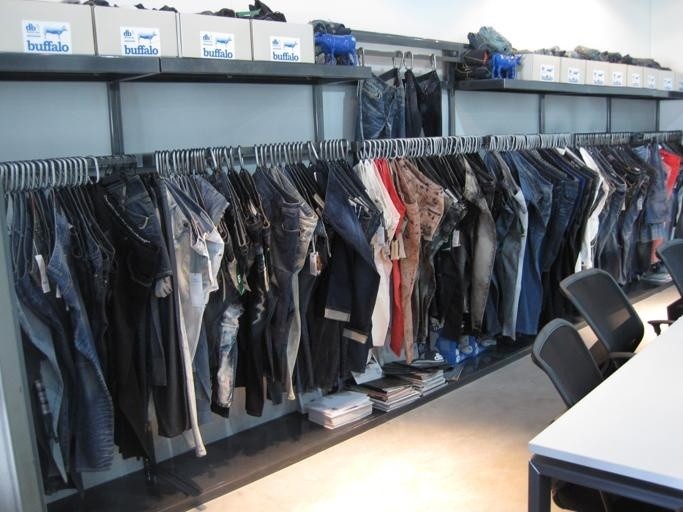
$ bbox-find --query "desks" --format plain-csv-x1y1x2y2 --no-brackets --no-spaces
528,315,683,512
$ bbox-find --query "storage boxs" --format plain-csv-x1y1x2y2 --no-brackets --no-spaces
176,12,252,60
250,18,315,65
1,1,95,58
92,5,178,58
510,55,683,92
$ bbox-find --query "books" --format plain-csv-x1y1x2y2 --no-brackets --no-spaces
299,352,462,432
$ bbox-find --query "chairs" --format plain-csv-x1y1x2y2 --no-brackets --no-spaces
559,268,674,372
531,318,604,408
655,237,683,326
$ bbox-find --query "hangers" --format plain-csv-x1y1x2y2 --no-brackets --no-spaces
1,153,138,193
152,135,481,176
486,131,682,153
